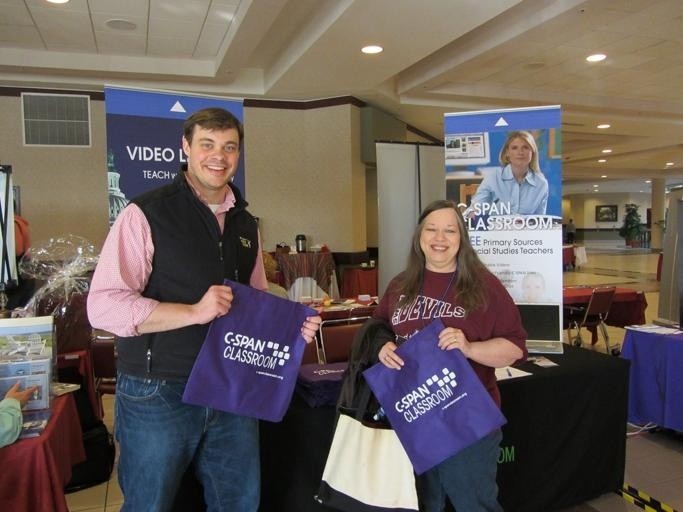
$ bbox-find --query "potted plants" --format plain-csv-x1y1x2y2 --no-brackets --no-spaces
618,203,643,248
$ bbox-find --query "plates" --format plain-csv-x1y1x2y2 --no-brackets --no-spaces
331,299,348,304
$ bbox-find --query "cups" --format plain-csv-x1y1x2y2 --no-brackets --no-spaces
295,235,306,253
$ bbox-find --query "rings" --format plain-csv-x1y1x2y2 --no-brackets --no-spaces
454,337,457,344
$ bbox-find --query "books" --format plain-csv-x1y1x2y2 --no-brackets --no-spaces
624,317,682,335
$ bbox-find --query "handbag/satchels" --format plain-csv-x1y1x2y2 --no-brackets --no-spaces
310,405,419,511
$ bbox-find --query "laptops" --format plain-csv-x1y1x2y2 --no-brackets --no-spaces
514,303,563,354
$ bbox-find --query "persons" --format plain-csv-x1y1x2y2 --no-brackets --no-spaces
1,380,38,447
471,130,552,221
364,202,529,512
565,219,577,243
86,108,324,509
520,272,546,306
